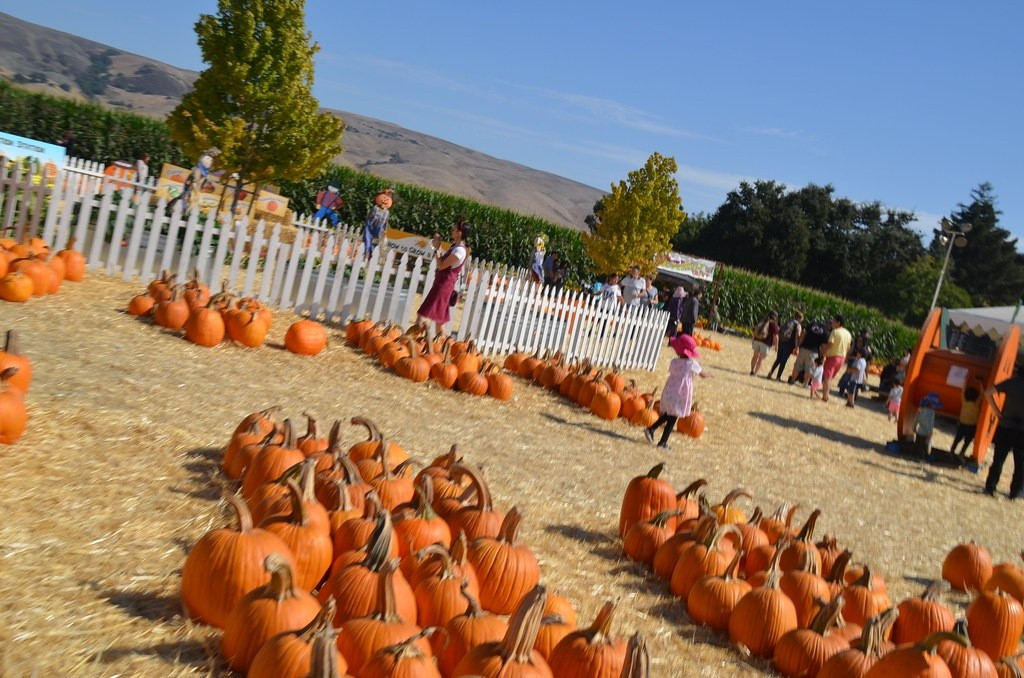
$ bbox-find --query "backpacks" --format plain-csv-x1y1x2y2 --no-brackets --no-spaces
754,320,774,339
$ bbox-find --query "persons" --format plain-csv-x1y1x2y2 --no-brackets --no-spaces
748,309,874,407
540,250,570,299
577,264,704,344
415,220,472,340
527,235,545,300
165,153,212,217
911,390,944,456
983,364,1024,500
950,374,985,465
643,334,710,451
362,188,393,262
309,181,344,228
132,152,150,195
878,348,913,425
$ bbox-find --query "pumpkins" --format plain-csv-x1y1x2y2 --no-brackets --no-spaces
619,462,1024,678
468,271,586,320
284,320,329,356
0,228,85,302
305,236,357,257
0,329,32,445
676,326,720,350
180,407,653,678
128,267,275,349
345,313,513,402
505,343,705,437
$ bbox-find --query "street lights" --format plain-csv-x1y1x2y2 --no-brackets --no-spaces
929,230,967,312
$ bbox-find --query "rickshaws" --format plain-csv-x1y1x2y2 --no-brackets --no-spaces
651,270,700,334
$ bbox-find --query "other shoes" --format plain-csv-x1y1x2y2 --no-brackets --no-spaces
644,428,655,443
658,441,673,451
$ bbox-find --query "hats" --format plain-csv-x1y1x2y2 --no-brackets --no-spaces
631,265,640,270
920,393,943,410
670,334,699,358
673,287,688,298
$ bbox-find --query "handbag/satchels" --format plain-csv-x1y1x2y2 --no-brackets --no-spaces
450,290,459,306
